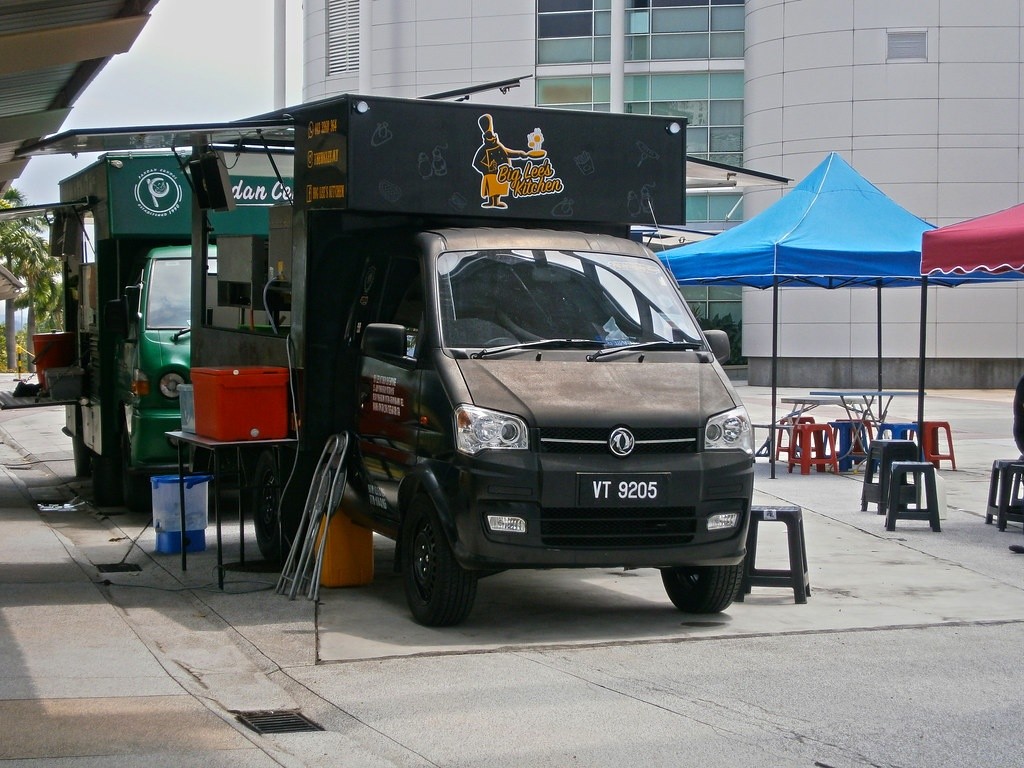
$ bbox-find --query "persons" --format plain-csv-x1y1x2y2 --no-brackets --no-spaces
1013,375,1024,456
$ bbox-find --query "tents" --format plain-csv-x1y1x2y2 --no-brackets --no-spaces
654,152,1024,480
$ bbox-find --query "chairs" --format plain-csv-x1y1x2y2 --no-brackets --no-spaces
273,430,351,602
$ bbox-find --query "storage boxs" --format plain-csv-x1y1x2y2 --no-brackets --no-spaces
154,529,207,555
150,474,212,533
43,366,86,402
176,384,196,435
190,363,289,440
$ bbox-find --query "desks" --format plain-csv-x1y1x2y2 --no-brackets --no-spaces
809,391,928,477
165,430,303,592
768,397,878,463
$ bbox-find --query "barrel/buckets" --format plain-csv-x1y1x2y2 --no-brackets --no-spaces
150,472,214,553
33,332,74,384
315,511,373,587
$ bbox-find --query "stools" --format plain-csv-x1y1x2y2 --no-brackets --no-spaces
985,455,1024,532
733,416,957,606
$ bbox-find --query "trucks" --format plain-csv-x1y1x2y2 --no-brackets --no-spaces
13,105,793,628
0,144,300,507
